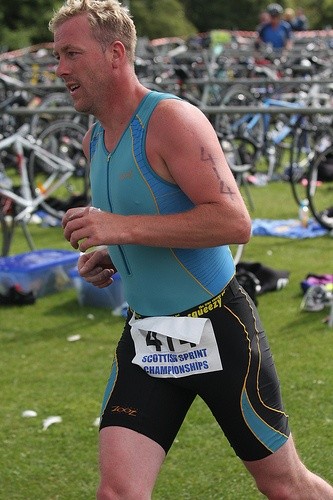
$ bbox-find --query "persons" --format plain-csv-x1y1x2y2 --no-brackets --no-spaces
47,0,333,500
258,7,307,30
256,4,292,52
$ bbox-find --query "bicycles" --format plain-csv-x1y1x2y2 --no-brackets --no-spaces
0,27,333,259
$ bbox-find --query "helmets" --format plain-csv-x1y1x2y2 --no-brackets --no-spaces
267,4,283,16
